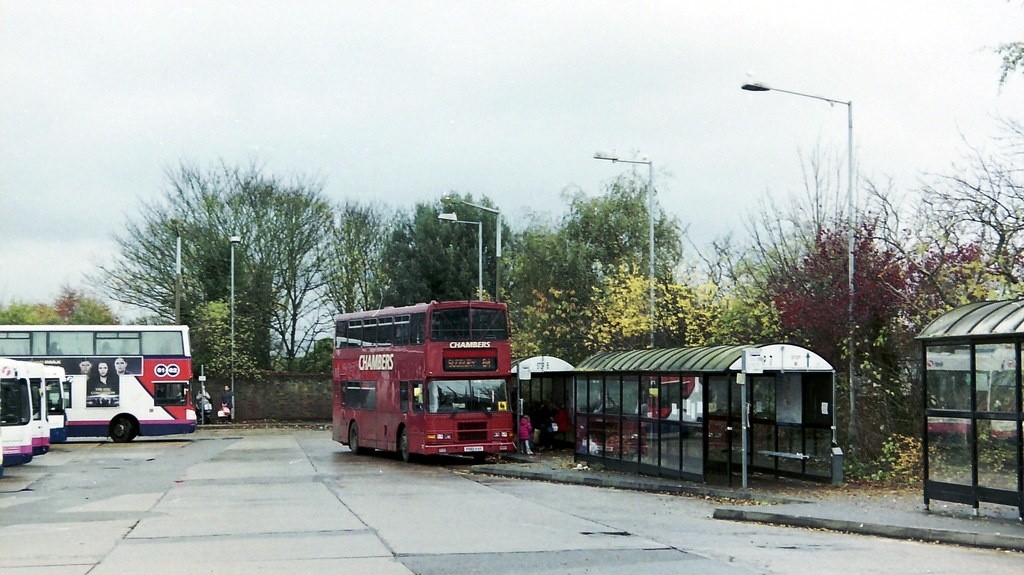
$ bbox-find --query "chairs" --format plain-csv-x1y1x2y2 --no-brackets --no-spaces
385,334,497,344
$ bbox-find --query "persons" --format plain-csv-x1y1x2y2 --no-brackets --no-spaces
536,400,569,452
222,385,232,424
520,416,533,455
79,357,130,396
195,390,212,424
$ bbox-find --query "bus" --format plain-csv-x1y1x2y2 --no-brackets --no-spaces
0,358,33,467
0,324,197,443
331,298,516,462
927,355,1017,438
576,378,704,460
45,365,69,445
9,359,50,457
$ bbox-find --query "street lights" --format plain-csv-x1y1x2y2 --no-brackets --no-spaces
440,197,503,302
437,213,484,302
228,236,242,423
169,216,182,325
594,152,654,350
741,81,858,425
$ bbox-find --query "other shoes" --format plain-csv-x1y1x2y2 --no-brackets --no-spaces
521,451,524,453
228,422,232,425
527,450,533,454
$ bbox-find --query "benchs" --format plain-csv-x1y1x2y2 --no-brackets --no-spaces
721,446,812,461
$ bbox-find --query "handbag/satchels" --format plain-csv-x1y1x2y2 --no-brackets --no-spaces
551,422,558,431
218,409,228,417
205,402,212,411
222,405,230,414
